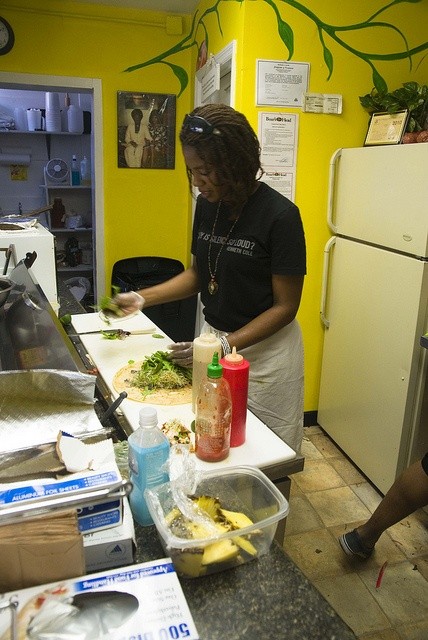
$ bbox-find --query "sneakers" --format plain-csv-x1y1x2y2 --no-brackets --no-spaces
338,527,374,563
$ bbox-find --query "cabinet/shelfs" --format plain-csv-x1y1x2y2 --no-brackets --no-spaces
43,164,96,305
68,307,306,549
0,130,83,161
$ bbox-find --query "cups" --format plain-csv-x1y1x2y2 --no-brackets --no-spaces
26,106,45,131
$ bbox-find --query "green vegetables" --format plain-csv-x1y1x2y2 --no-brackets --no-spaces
140,350,192,386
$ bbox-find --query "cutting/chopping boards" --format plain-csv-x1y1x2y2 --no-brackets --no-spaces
70,306,296,480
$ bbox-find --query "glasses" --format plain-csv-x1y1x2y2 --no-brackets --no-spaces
183,113,237,149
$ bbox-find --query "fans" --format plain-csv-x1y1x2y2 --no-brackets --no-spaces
44,157,72,185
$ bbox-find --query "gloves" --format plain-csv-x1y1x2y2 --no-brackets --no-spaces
103,291,145,321
166,342,193,369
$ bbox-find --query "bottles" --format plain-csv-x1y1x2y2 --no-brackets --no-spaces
80,156,91,186
72,154,80,185
126,407,171,527
218,346,250,449
194,351,232,462
191,325,223,414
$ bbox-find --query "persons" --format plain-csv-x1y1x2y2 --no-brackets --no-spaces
142,109,167,168
105,104,306,454
339,453,428,558
124,109,152,168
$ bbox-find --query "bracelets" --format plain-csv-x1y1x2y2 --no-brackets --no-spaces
221,335,230,356
131,291,146,309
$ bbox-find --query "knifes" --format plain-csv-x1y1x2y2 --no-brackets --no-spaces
66,327,156,337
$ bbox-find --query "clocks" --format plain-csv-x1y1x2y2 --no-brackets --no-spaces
0,15,14,55
